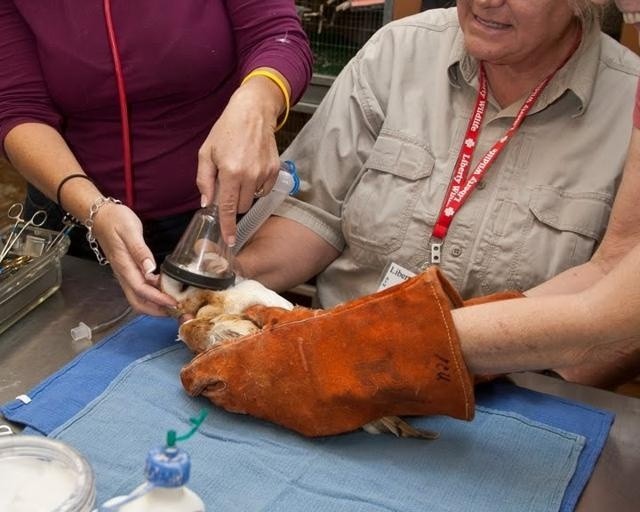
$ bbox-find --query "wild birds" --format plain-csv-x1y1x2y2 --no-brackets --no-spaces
158,251,440,441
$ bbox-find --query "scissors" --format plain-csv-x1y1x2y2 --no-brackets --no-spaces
0,203,48,263
45,213,84,254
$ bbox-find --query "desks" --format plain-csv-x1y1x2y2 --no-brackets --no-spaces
1,250,640,512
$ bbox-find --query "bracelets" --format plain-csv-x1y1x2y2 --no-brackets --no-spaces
240,69,292,132
83,196,122,267
56,173,97,229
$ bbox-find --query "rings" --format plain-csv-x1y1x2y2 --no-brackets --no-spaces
254,190,264,198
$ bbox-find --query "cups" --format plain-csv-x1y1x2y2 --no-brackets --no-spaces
0,436,97,512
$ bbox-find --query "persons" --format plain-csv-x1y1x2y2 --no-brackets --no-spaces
0,0,315,321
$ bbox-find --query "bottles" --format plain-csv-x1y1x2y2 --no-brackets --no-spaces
99,410,208,512
